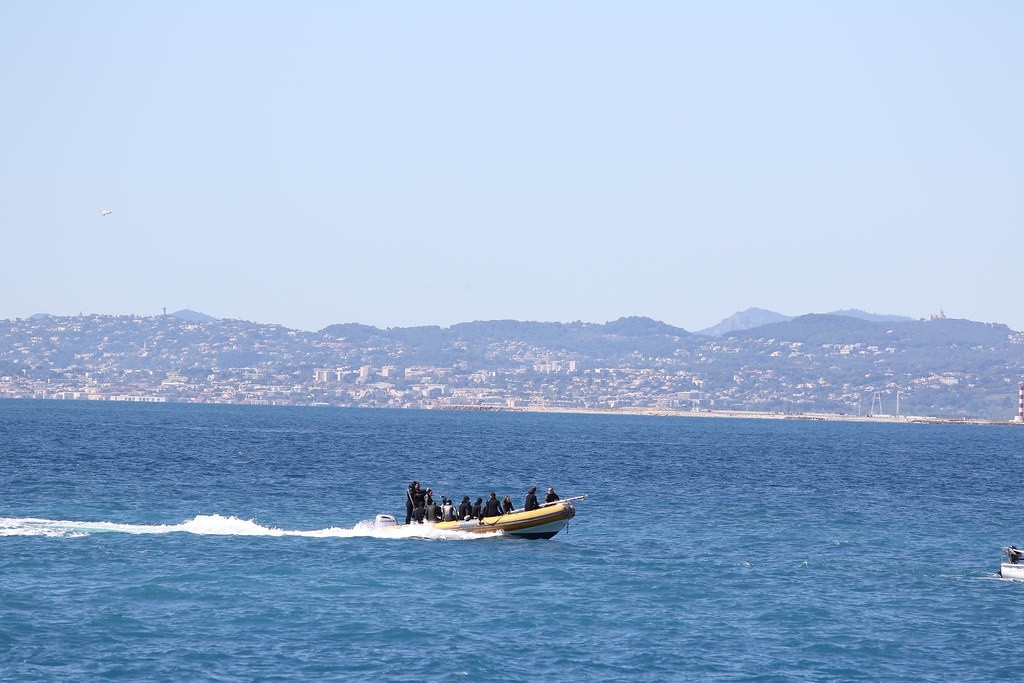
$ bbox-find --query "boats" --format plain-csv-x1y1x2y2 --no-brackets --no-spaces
375,493,589,540
995,544,1024,580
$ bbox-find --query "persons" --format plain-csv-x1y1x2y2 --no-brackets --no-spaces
502,496,515,514
525,486,543,511
544,487,559,508
406,481,506,524
